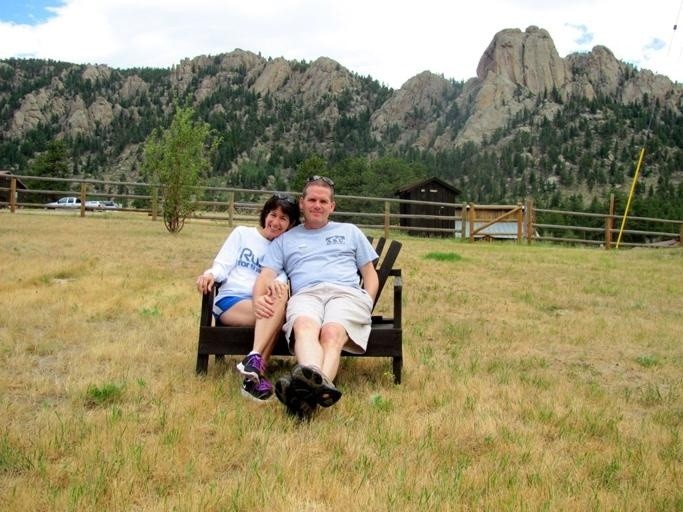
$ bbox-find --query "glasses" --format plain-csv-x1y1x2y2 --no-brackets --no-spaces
308,175,335,186
274,193,298,205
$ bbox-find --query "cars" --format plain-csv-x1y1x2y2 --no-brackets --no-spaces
44,196,119,211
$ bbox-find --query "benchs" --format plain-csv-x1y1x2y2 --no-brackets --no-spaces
196,269,402,385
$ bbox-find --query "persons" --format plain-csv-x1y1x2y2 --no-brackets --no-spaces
197,193,301,403
251,178,380,418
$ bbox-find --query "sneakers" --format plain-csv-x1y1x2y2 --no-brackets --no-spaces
239,376,273,401
237,353,265,384
275,365,342,417
